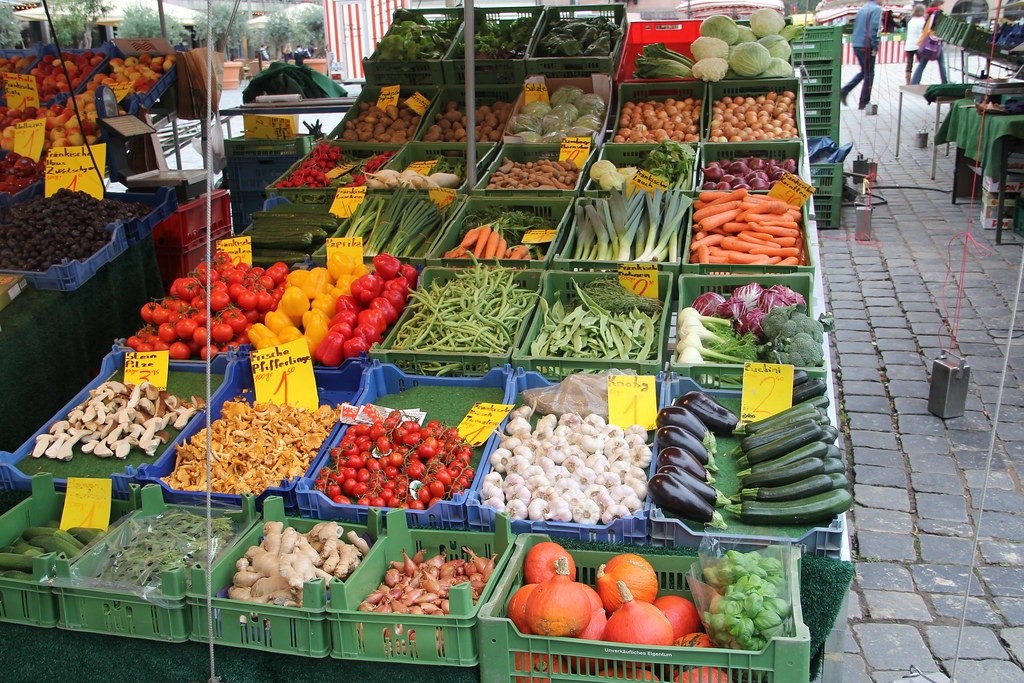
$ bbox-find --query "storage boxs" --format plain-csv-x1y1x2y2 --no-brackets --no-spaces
153,189,232,293
790,22,844,231
614,19,702,96
503,71,613,150
980,170,1023,230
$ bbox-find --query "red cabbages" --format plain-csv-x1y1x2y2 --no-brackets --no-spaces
691,282,807,342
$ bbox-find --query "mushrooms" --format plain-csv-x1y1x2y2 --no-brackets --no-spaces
159,401,349,495
32,381,208,460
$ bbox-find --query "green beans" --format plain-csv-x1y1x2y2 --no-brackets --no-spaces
390,250,663,377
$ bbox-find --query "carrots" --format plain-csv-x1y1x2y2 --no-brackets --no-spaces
443,208,557,260
689,188,806,265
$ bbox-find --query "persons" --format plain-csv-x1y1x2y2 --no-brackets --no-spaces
840,0,883,111
882,9,902,33
898,13,907,27
259,44,269,61
909,0,954,86
904,3,927,85
282,44,315,66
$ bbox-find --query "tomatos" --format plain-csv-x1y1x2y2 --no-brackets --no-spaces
126,252,291,361
313,406,475,510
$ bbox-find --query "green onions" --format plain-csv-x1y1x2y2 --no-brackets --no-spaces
344,182,459,258
571,184,693,272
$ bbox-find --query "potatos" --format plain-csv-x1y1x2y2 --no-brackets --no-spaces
341,97,513,144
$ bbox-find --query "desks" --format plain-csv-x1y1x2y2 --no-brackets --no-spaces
935,100,1023,247
218,95,356,192
895,84,958,180
0,228,164,452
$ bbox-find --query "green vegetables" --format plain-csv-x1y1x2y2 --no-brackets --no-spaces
375,9,624,62
632,43,696,79
98,511,235,589
642,140,697,190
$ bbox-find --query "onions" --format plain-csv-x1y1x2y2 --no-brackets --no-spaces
700,155,799,190
357,546,499,653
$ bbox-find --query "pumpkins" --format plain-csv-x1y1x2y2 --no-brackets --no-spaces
508,542,727,683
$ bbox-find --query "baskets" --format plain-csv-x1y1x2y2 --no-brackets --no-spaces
935,16,1001,58
1013,196,1024,239
0,4,843,683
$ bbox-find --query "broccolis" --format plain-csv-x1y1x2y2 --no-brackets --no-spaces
757,305,824,368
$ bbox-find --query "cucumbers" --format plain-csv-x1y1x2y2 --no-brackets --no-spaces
0,519,107,581
230,212,339,269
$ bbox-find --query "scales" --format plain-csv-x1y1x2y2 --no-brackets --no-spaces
95,113,212,204
973,78,1024,108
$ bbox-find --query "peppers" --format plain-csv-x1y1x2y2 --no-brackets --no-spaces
247,253,419,367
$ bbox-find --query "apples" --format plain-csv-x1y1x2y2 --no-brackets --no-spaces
0,51,176,197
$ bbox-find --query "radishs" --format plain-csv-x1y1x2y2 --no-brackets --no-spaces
359,156,467,189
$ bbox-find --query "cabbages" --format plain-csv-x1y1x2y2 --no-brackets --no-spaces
690,8,808,82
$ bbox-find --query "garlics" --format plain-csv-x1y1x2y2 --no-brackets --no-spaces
479,404,653,525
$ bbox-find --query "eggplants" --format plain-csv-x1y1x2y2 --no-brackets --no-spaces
647,391,742,531
